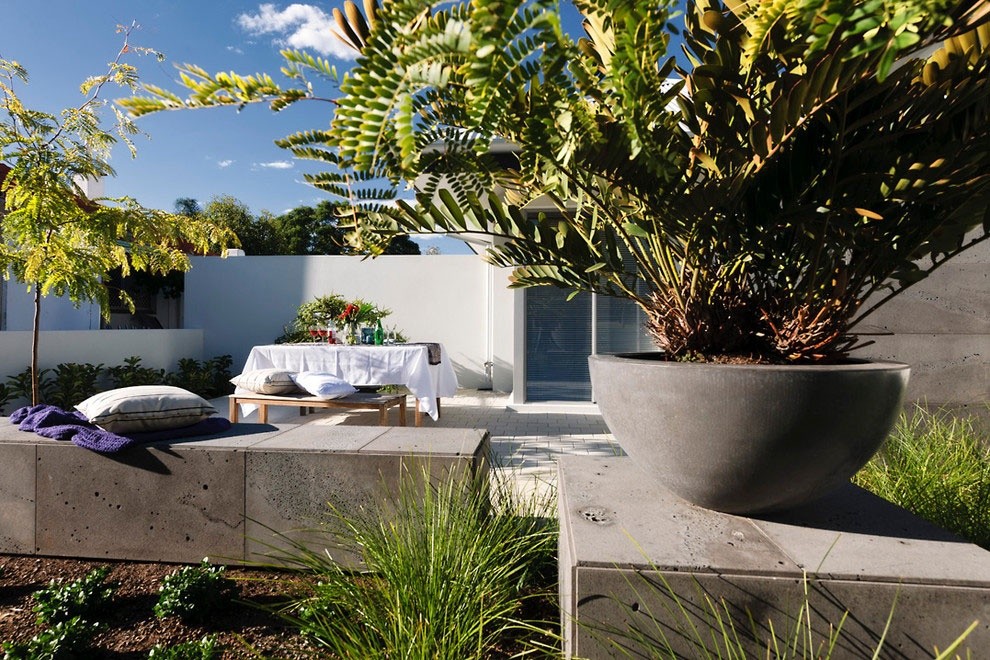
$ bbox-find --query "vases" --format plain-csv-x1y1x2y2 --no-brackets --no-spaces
347,321,355,346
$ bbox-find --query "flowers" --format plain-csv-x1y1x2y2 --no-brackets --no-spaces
339,304,360,320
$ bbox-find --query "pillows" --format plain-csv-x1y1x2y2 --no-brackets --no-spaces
288,370,360,400
74,385,218,440
229,369,300,395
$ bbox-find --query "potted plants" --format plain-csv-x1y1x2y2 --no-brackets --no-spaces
258,0,990,515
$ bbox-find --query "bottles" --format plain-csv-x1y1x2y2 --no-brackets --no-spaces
328,317,336,344
374,319,383,346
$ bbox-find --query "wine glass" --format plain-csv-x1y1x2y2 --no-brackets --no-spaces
309,326,327,343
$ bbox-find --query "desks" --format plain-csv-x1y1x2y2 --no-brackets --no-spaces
234,342,458,428
229,392,406,427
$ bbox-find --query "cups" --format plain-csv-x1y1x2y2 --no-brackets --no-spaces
383,329,396,346
